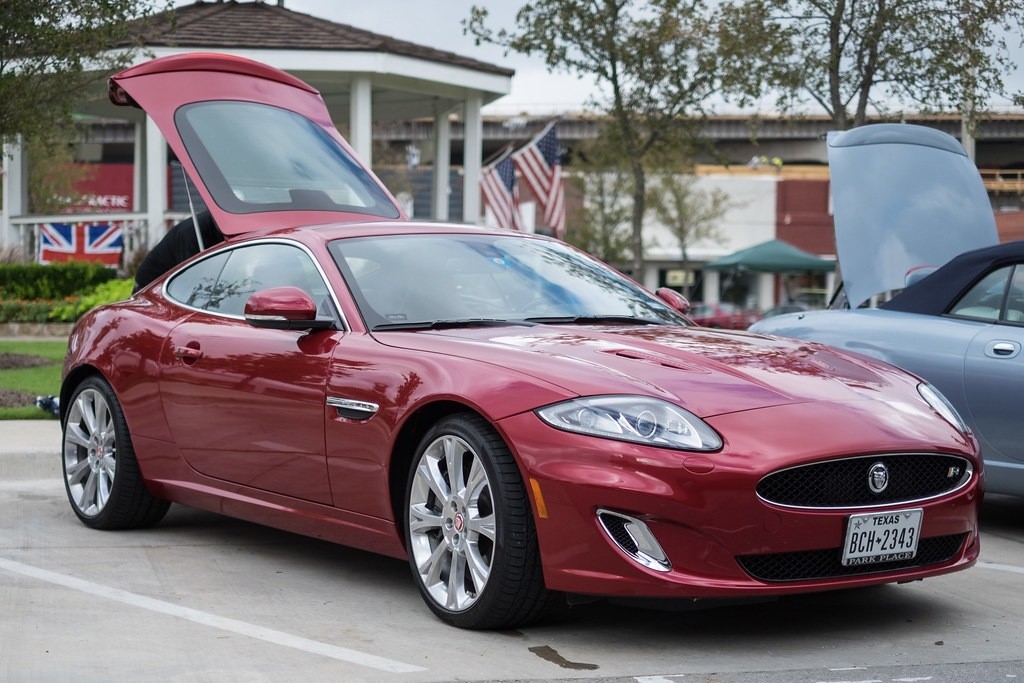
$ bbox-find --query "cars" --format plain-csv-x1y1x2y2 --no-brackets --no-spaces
689,303,760,330
757,303,809,318
745,122,1024,509
58,53,987,631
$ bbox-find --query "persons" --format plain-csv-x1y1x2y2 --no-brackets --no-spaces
132,188,245,298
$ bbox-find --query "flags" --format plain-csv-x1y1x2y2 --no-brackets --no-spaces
479,121,565,242
37,219,124,265
